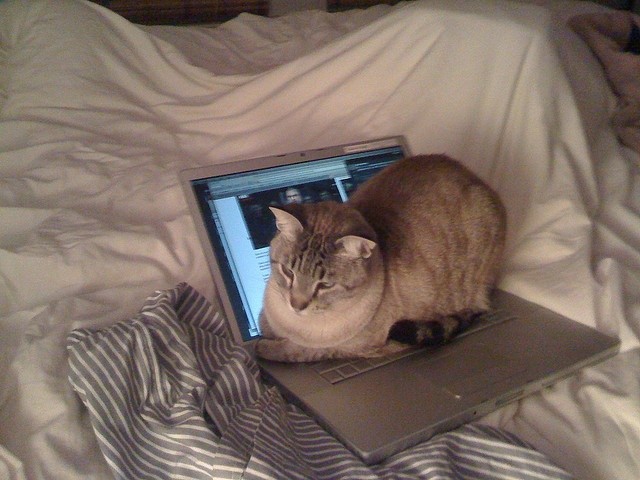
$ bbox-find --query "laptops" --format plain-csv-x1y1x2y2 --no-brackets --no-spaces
180,135,620,463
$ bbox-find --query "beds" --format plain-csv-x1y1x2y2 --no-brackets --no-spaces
2,4,640,478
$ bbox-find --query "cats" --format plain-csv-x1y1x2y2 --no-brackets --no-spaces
251,152,511,364
